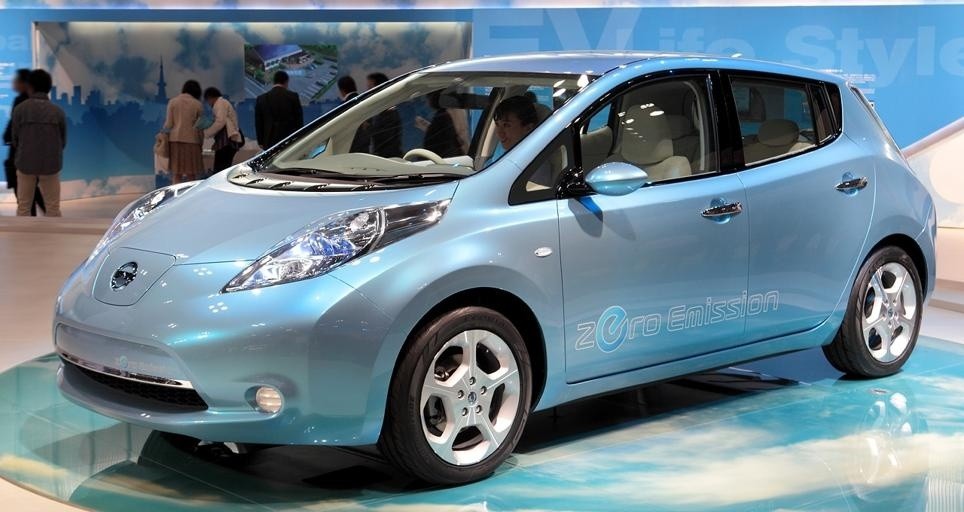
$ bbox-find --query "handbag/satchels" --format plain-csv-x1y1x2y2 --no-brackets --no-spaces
155,130,169,156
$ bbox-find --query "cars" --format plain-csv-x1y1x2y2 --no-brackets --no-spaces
54,49,936,487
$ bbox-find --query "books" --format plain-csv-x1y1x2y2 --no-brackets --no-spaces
414,114,430,134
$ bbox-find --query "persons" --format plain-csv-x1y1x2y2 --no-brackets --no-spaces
415,88,463,161
1,68,47,216
10,69,65,216
337,75,371,154
449,95,471,156
488,91,561,188
198,87,245,174
254,70,303,152
366,72,402,158
162,79,204,185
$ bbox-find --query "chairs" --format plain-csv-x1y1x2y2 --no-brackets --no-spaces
527,101,813,185
